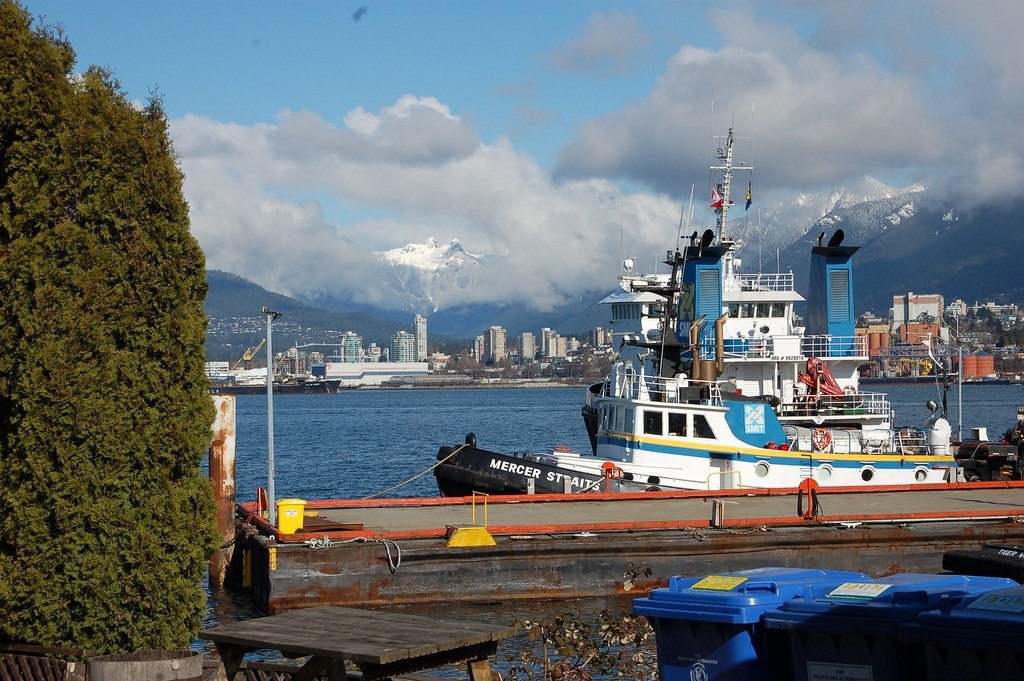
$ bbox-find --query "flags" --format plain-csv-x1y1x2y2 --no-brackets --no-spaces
711,190,722,208
745,179,752,210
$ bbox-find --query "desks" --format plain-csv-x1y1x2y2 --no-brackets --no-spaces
198,605,516,681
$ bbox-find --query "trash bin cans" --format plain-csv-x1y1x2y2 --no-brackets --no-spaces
760,572,1019,681
275,497,307,535
630,566,875,681
894,579,1024,681
942,543,1023,582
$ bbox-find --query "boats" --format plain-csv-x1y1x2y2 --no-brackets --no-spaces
432,117,1024,495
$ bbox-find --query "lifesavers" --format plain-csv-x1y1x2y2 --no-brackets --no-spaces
813,428,831,450
